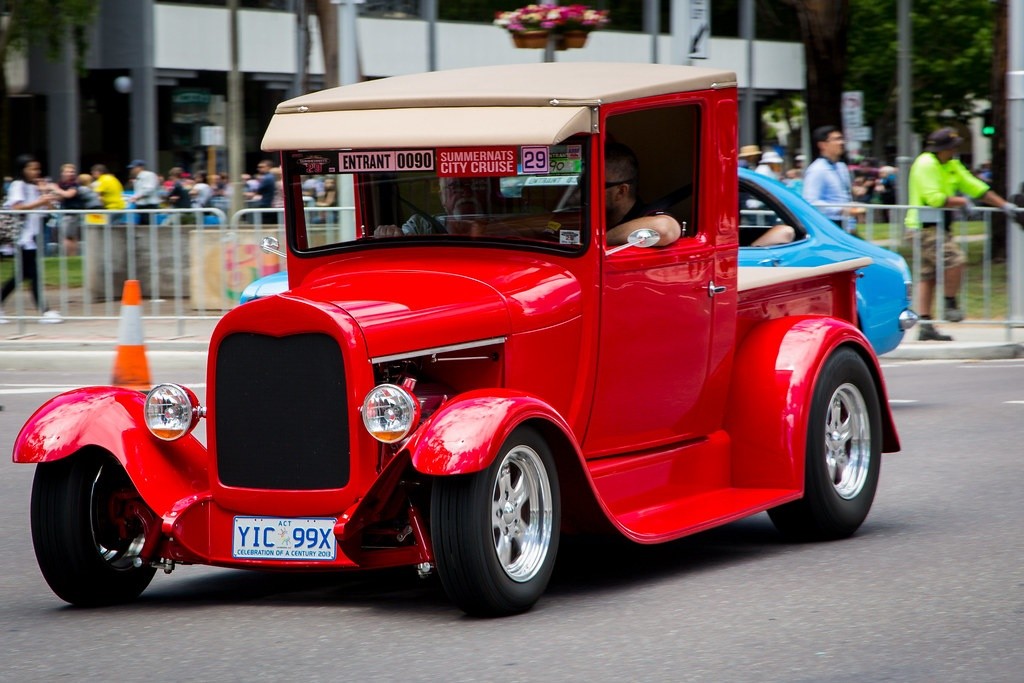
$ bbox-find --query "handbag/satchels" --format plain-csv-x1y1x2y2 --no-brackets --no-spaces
0,214,26,244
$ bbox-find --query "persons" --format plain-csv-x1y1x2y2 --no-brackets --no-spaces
90,164,127,223
373,175,489,238
854,166,897,223
128,160,160,225
902,128,1017,343
300,175,338,223
802,125,860,236
0,156,63,324
51,163,82,258
160,160,284,226
476,143,683,247
738,144,805,182
753,225,795,247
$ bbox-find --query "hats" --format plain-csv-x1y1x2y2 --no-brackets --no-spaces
758,151,784,164
737,145,762,157
924,129,964,152
128,160,145,168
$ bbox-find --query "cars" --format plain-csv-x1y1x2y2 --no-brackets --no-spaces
236,162,920,357
4,58,903,621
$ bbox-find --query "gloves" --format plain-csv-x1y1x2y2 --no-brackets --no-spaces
1002,203,1019,218
963,201,975,216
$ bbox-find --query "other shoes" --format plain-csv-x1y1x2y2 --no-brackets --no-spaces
944,309,964,322
38,310,65,324
919,326,952,342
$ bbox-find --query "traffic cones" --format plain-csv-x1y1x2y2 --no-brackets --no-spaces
109,278,152,394
